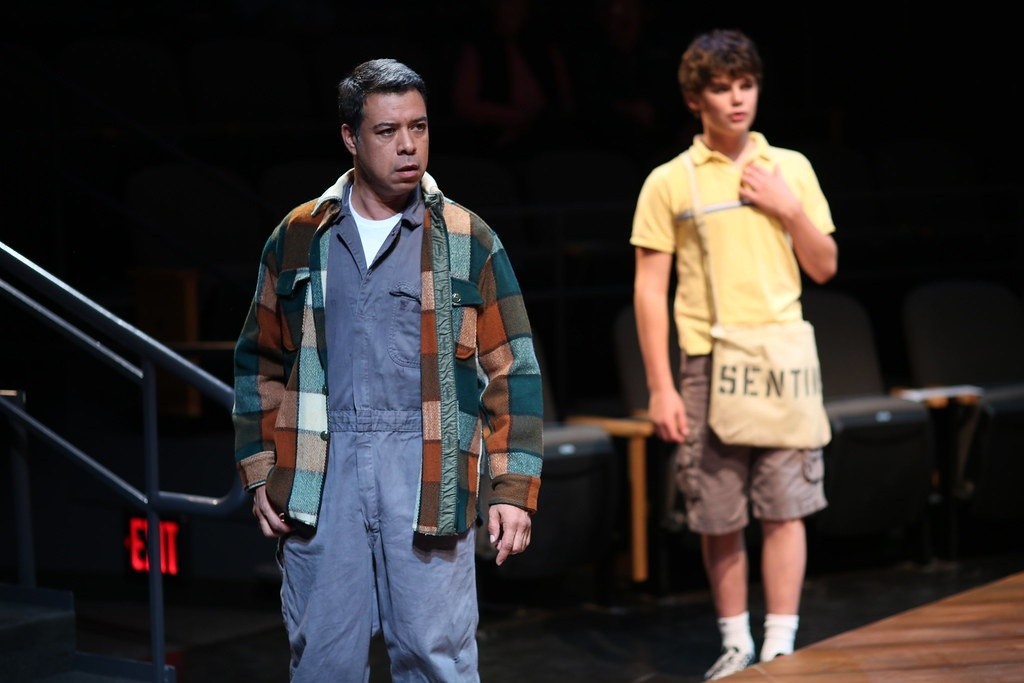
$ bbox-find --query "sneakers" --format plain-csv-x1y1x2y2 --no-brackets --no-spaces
705,646,756,682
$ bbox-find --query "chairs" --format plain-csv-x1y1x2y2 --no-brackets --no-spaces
492,280,1022,582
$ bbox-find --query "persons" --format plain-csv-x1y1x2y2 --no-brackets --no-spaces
629,29,837,682
231,58,544,683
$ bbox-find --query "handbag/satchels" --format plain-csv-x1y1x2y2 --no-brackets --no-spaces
708,322,831,449
